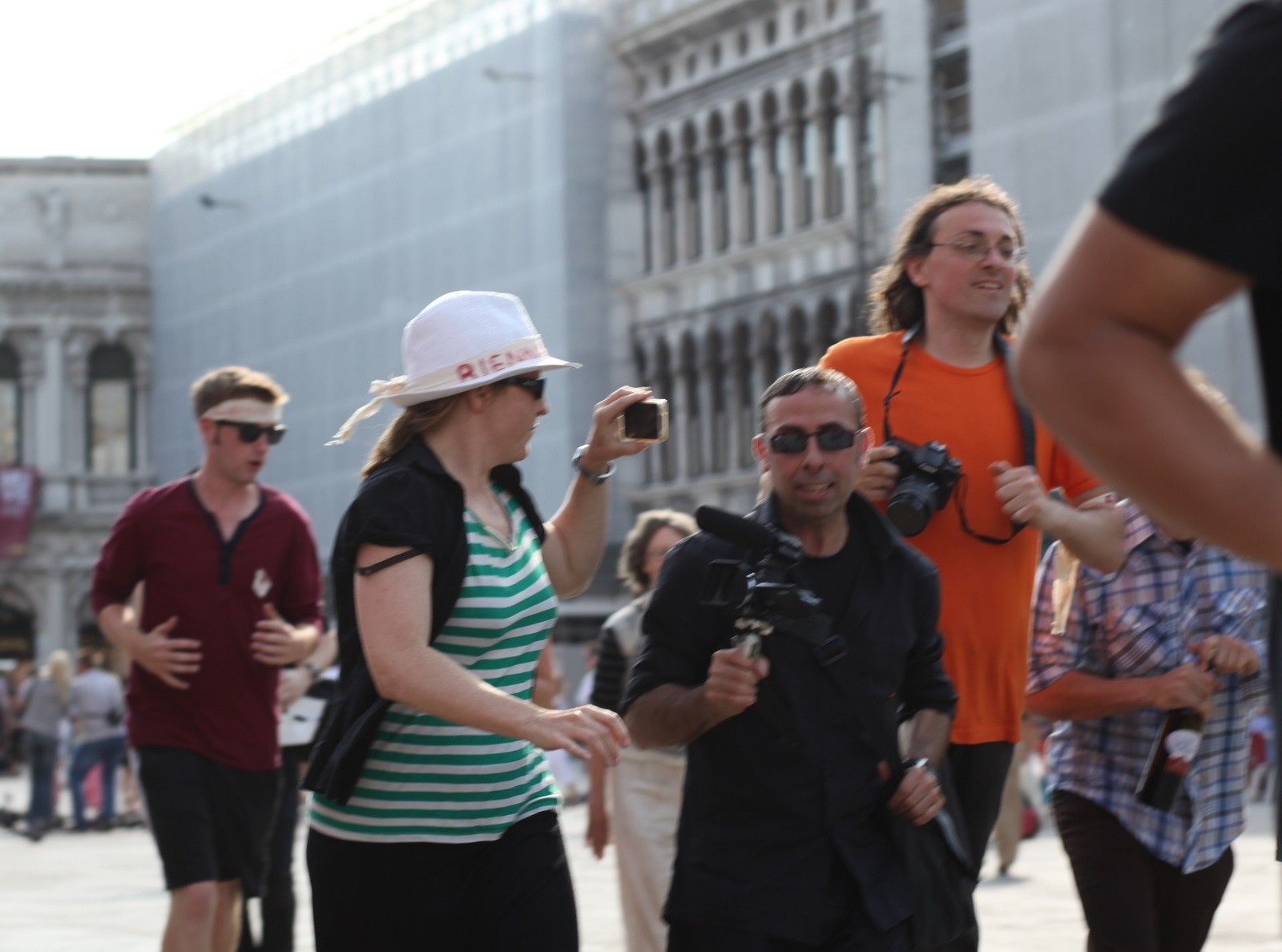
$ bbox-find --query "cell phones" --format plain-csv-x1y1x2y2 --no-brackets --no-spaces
617,398,669,445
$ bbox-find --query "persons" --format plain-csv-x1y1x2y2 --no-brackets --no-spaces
574,641,602,705
618,362,960,952
0,637,150,840
586,507,700,952
816,182,1128,888
1013,0,1281,861
234,608,340,952
297,291,653,952
1023,368,1273,952
86,368,326,952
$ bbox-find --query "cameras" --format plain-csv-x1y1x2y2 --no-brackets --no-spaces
874,436,965,538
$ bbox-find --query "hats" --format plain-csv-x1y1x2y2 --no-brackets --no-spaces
324,290,583,445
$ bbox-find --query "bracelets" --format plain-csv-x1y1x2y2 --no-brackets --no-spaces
304,662,320,679
898,755,941,782
571,444,616,485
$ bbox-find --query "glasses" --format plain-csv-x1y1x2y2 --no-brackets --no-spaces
492,376,546,400
644,545,669,558
764,425,864,453
216,420,287,444
917,236,1024,262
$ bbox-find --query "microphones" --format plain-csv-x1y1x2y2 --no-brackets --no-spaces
693,505,780,558
